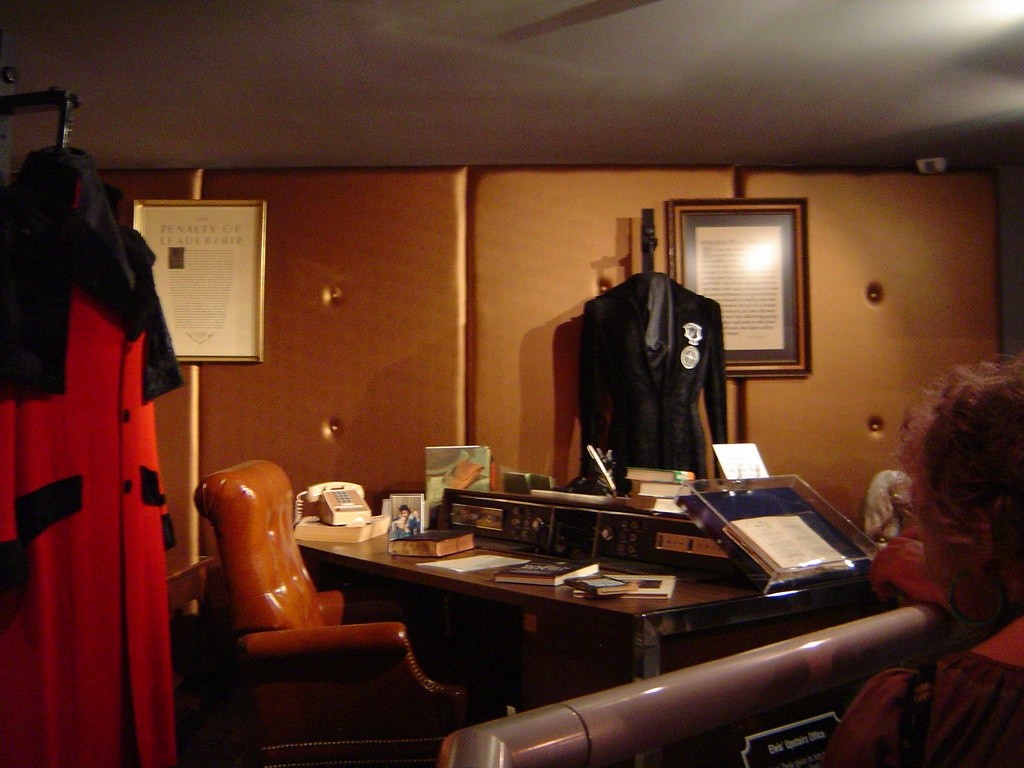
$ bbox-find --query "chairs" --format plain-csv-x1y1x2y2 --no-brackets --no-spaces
192,456,470,768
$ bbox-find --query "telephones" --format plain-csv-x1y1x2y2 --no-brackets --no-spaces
305,480,372,526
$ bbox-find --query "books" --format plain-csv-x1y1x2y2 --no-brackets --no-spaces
293,514,390,543
566,573,633,597
496,555,599,587
625,467,695,515
388,529,475,557
573,574,678,599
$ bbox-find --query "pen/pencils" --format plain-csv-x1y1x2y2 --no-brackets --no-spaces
585,444,618,496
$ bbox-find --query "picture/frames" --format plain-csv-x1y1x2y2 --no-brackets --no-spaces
668,197,812,377
388,493,425,540
134,199,268,364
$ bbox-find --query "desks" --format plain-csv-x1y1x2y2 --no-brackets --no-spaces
296,526,896,768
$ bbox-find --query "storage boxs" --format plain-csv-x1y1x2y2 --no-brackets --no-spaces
675,473,882,595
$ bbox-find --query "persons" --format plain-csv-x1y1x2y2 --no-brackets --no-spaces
392,504,422,541
835,355,1024,767
